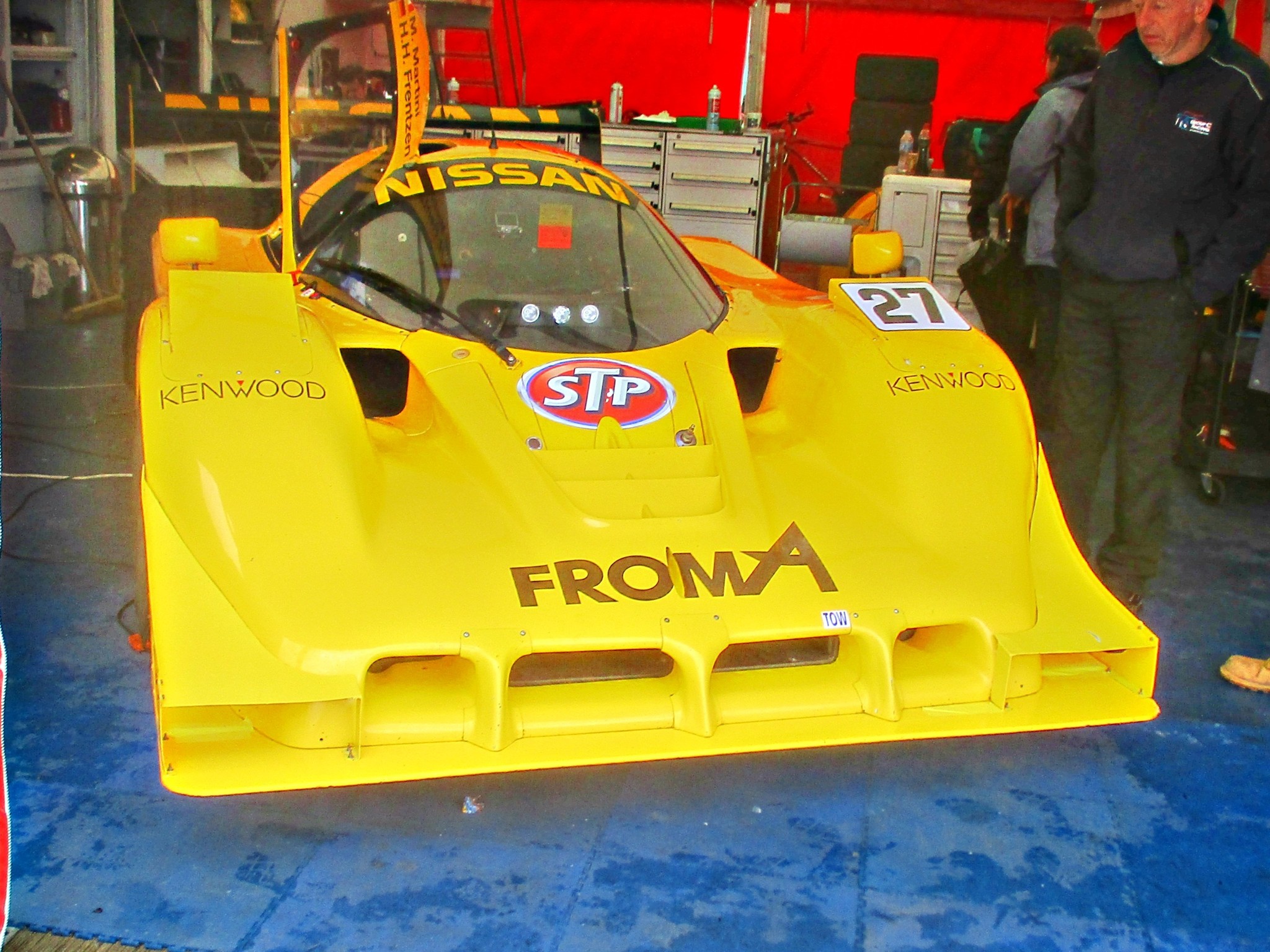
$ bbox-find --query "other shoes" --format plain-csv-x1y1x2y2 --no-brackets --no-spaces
1220,654,1270,694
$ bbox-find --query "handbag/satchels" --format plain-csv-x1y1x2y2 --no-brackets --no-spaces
941,117,1010,179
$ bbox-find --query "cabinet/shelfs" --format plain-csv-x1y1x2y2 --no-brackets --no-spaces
0,0,119,267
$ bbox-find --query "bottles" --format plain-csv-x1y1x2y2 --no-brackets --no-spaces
913,123,931,176
896,130,914,175
446,77,460,106
608,81,623,124
707,85,721,132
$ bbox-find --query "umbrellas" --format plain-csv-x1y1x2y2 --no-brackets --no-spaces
957,193,1063,433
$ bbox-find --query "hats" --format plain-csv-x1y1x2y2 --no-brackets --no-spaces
213,70,255,96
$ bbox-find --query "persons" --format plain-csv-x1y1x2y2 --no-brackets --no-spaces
966,26,1103,433
1220,654,1270,693
1048,0,1270,617
321,60,392,102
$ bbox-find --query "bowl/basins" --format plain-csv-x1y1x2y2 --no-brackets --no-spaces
30,30,57,47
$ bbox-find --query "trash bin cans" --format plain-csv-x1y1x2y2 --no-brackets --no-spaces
40,147,125,304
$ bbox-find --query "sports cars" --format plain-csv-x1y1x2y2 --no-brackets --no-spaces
131,1,1166,799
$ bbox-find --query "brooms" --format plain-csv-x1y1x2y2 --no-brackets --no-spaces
1,72,126,316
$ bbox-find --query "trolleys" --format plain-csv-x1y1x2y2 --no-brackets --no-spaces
1174,271,1270,506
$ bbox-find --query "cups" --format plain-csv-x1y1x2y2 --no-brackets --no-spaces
747,112,762,132
49,101,72,133
